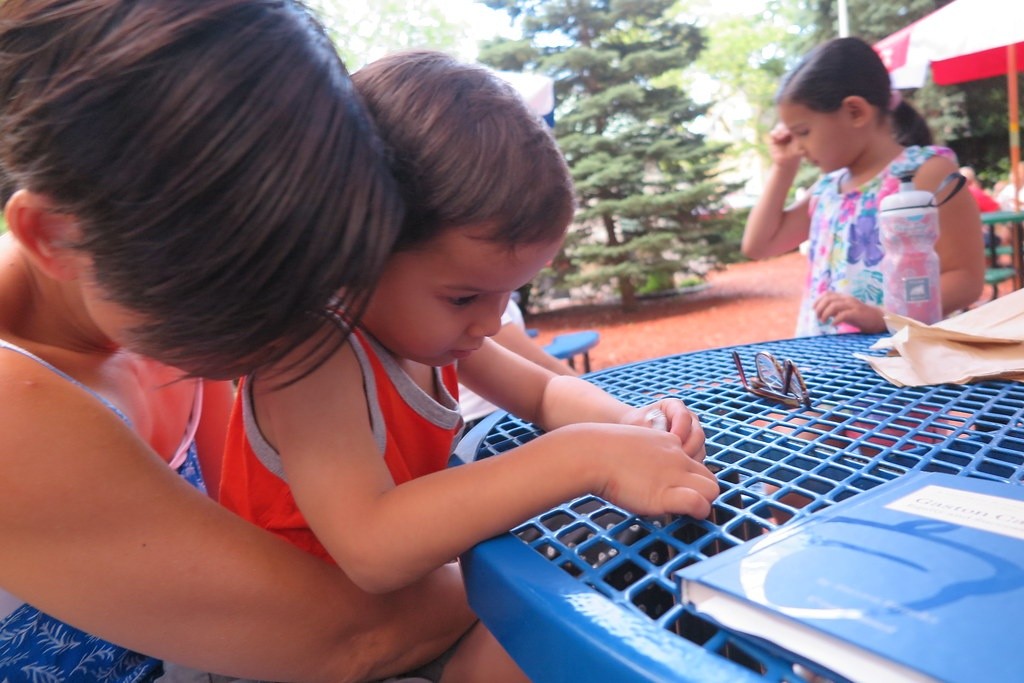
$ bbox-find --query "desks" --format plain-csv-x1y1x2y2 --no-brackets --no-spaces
445,327,1024,683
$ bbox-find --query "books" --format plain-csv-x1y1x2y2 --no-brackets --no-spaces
676,471,1022,683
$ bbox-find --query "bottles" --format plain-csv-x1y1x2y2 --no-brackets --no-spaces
877,172,965,335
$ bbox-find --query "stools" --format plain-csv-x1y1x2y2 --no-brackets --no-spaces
542,331,600,373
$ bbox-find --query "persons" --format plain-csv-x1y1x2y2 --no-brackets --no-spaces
740,38,986,528
163,51,720,683
954,164,1023,269
1,1,527,683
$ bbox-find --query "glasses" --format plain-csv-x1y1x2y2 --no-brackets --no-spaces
732,350,811,407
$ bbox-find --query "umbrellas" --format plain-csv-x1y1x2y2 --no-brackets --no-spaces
873,0,1024,206
